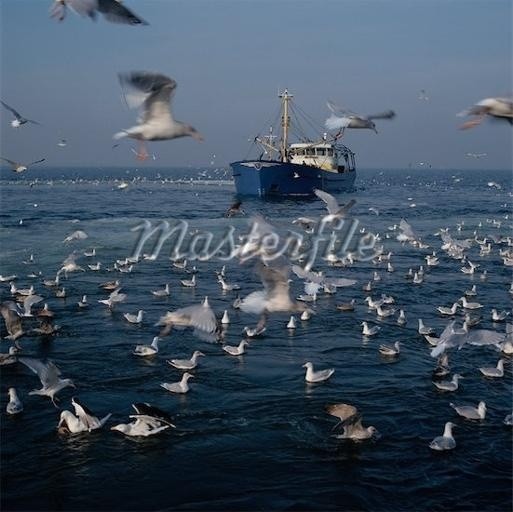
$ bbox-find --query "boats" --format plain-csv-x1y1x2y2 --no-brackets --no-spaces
229,88,356,201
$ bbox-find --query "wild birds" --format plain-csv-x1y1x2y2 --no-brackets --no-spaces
48,0,150,26
0,71,513,451
